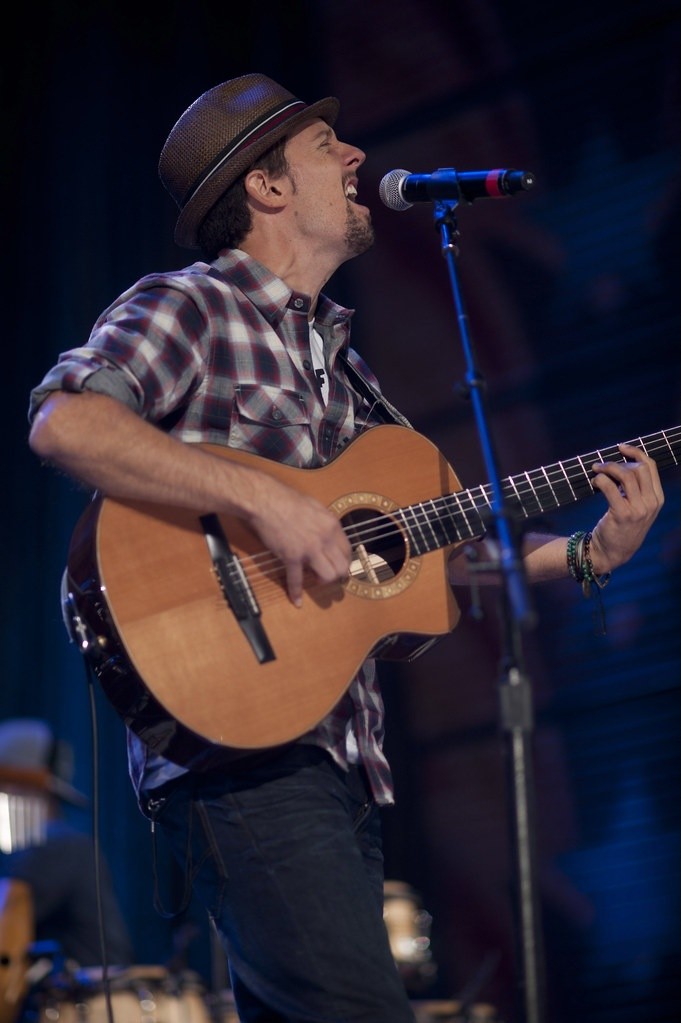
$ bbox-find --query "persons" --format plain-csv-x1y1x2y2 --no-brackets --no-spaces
28,72,665,1023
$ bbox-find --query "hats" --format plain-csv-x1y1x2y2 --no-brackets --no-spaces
154,70,345,250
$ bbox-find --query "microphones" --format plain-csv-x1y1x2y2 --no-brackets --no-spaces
379,168,536,211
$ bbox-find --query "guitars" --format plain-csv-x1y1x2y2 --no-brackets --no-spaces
64,420,681,777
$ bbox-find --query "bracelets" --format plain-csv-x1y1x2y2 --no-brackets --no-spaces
567,531,611,637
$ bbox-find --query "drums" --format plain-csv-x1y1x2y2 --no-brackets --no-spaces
35,964,213,1023
376,879,431,985
411,1000,496,1023
